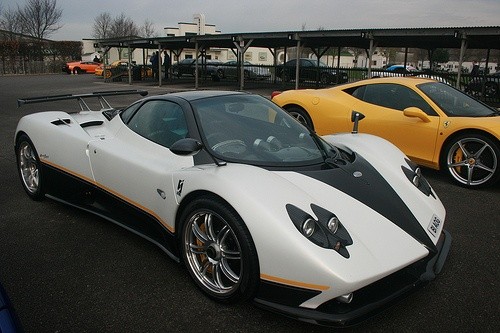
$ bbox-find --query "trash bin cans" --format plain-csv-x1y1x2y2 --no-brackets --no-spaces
132,66,142,81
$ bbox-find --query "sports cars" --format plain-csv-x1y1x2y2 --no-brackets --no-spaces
269,76,500,188
13,89,450,331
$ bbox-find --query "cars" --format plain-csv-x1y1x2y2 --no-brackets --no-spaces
369,64,452,87
276,58,348,84
96,59,153,78
468,71,500,98
171,59,221,78
212,60,271,80
62,60,103,75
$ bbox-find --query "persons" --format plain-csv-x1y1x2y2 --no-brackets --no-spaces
163,51,170,79
93,55,99,62
469,59,480,81
150,51,162,80
